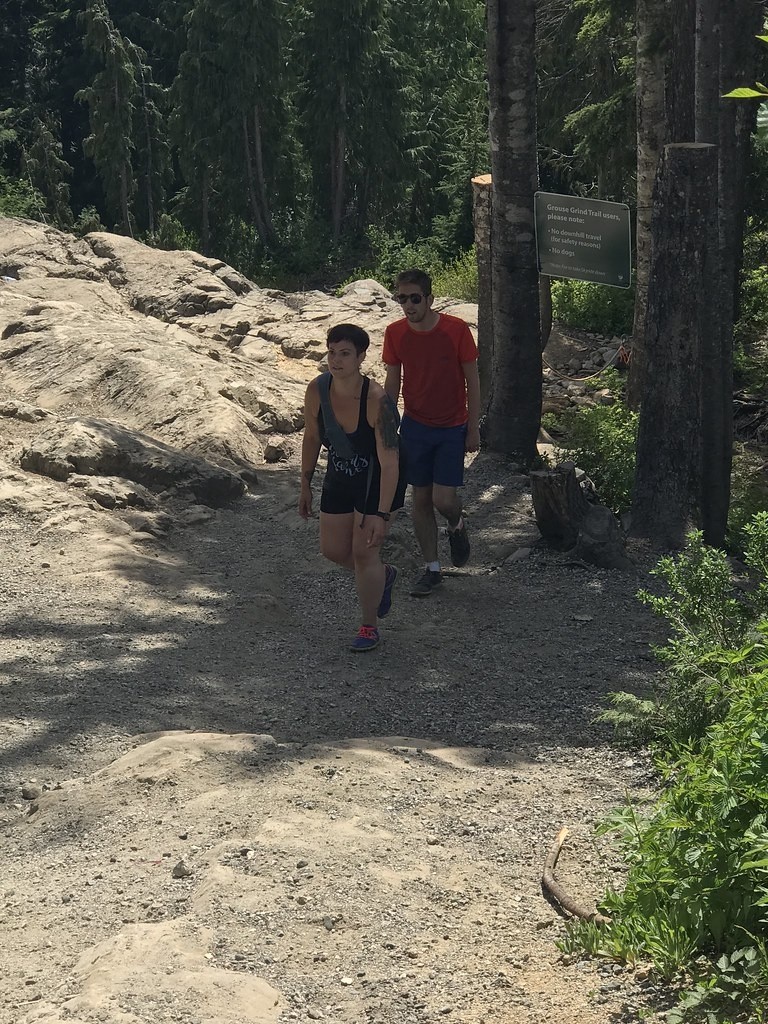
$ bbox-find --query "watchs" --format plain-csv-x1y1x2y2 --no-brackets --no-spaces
375,510,391,521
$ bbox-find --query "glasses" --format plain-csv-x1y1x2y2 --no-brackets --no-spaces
397,293,426,304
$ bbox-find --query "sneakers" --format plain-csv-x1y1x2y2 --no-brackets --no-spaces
377,564,398,619
409,562,443,594
447,517,470,567
349,624,380,651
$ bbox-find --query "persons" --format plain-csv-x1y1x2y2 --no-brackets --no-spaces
299,324,403,651
382,269,481,597
